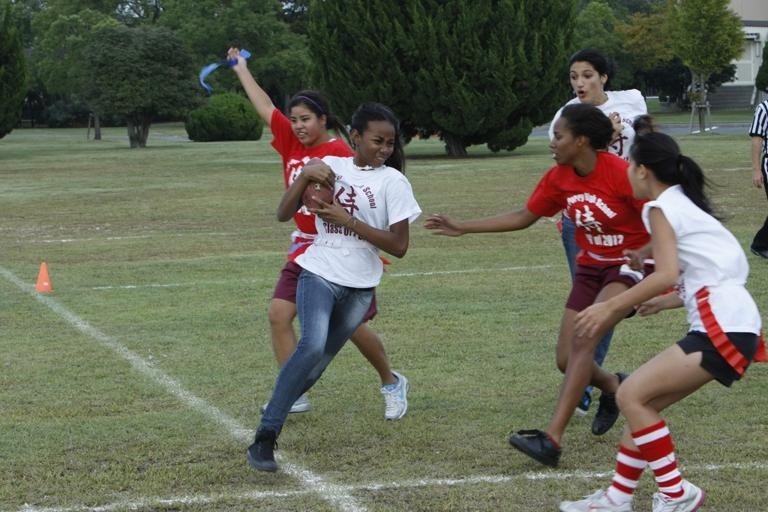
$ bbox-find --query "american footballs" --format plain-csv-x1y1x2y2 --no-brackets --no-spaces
301,158,333,209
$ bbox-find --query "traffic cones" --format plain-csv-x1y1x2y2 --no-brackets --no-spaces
34,262,54,292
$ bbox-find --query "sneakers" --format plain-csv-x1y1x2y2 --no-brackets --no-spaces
509,428,564,469
650,479,706,512
246,427,279,471
379,369,409,422
556,488,635,512
258,392,314,417
591,371,633,436
575,391,592,417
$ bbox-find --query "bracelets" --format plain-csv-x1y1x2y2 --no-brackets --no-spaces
344,216,359,229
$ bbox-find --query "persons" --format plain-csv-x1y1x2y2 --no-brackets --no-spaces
543,43,654,420
225,43,409,424
553,131,765,511
747,99,768,200
245,98,424,473
419,102,659,468
